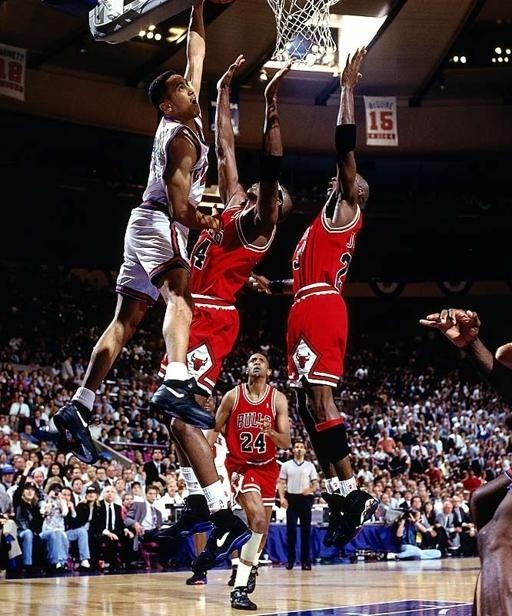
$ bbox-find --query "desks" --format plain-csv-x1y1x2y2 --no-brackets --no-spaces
163,522,406,572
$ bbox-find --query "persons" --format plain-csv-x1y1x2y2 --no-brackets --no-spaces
153,48,301,579
212,337,328,594
2,310,189,578
53,0,219,465
332,348,512,560
412,307,512,614
196,353,292,616
248,48,380,552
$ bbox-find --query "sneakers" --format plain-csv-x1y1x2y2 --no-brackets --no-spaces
157,496,258,611
53,404,98,465
150,384,215,429
321,488,378,547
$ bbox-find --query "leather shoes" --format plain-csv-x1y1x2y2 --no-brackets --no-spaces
302,563,311,570
286,561,294,569
15,566,100,579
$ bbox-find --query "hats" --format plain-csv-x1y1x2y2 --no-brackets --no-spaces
43,477,63,493
86,486,97,492
2,467,16,474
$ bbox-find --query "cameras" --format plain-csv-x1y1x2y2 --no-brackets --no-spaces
53,492,59,497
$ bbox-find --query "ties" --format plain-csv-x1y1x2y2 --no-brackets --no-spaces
108,504,112,530
